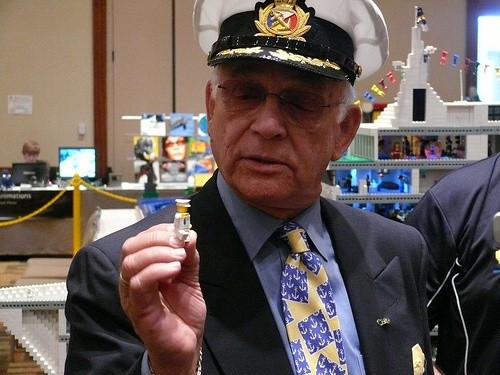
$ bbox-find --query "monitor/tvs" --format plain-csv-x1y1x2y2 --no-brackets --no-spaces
12,162,49,186
59,147,97,180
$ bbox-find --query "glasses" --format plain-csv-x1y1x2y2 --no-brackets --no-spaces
214,79,353,127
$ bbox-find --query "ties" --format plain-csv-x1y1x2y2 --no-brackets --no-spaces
268,223,349,375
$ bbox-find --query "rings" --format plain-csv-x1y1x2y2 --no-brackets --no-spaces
119,270,130,288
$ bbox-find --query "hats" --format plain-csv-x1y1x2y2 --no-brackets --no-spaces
192,0,390,83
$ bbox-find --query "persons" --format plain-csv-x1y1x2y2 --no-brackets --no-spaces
22,138,48,164
402,152,500,375
61,0,436,375
